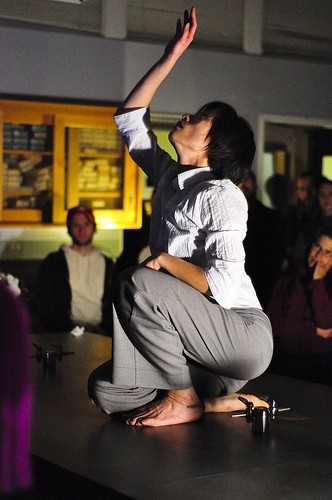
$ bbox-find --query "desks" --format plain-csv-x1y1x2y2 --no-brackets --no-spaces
29,332,332,500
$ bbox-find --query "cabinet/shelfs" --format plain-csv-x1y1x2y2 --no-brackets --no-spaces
1,99,143,230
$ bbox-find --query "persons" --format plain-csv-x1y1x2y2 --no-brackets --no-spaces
1,275,34,500
35,204,116,338
265,227,332,390
232,166,332,303
88,1,274,426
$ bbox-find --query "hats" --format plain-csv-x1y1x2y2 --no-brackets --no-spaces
68,206,93,222
